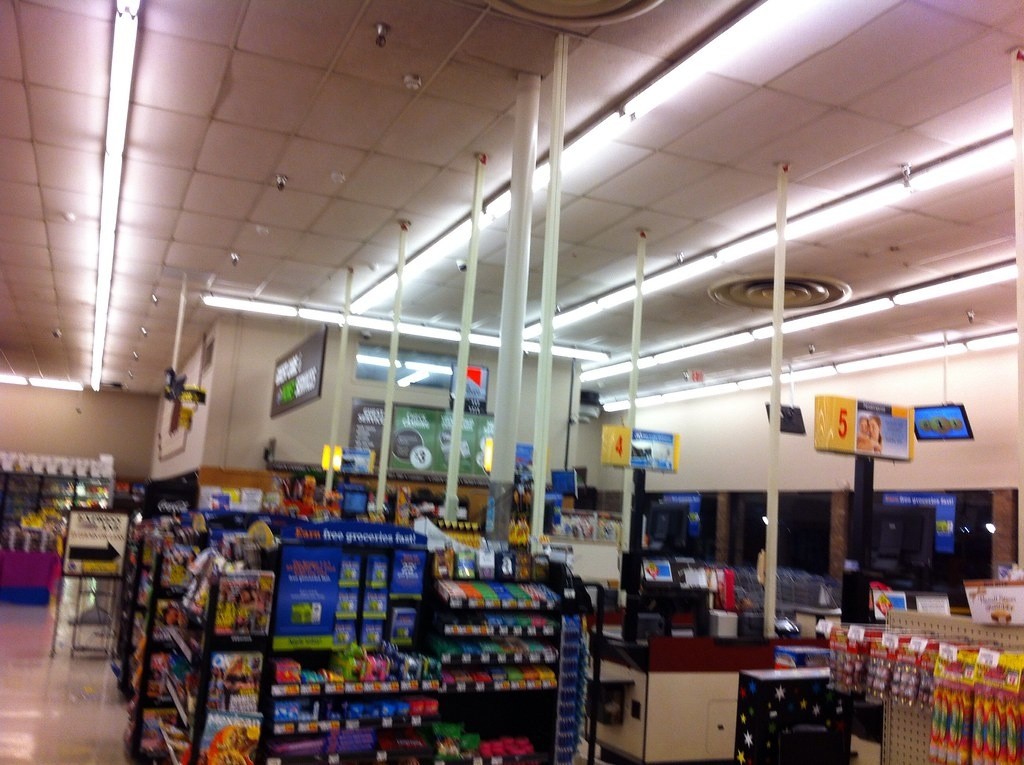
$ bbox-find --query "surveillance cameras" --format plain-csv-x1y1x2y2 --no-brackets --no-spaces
457,260,467,271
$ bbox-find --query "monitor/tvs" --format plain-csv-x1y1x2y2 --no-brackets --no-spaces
914,402,973,441
765,404,805,435
643,491,940,592
554,468,579,498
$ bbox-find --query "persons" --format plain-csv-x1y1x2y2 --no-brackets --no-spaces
857,415,882,452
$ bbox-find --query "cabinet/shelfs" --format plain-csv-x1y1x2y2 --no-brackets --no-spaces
116,522,153,700
257,542,430,765
428,552,566,765
151,570,278,765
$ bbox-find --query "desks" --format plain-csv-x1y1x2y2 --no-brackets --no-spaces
0,548,62,595
